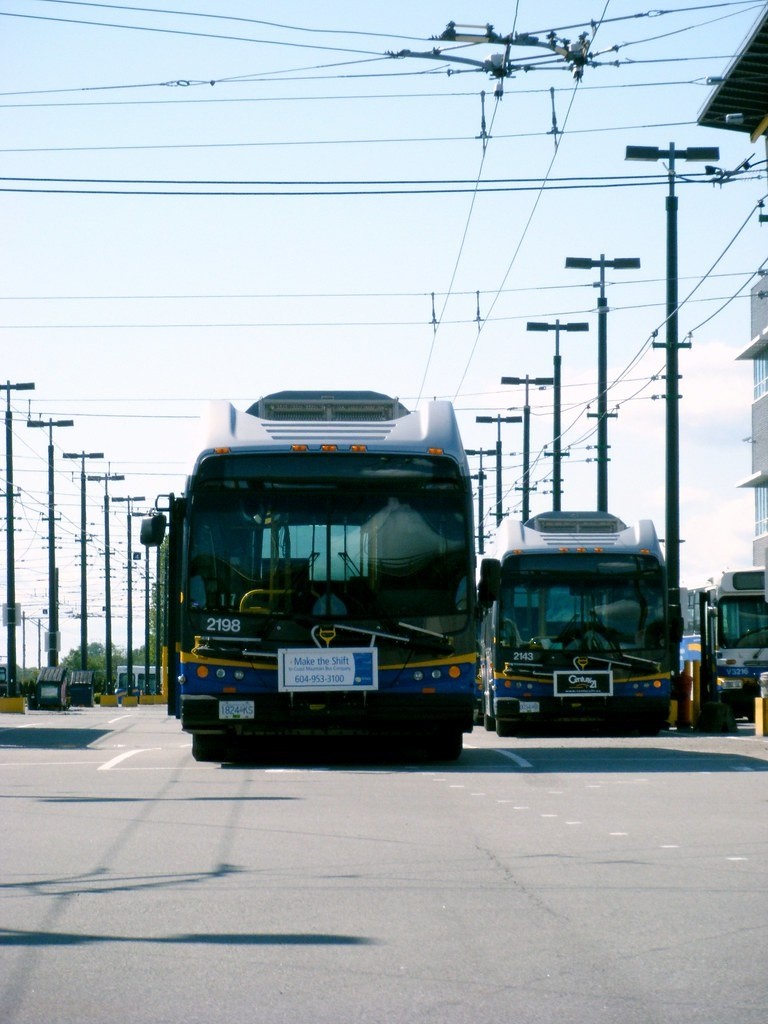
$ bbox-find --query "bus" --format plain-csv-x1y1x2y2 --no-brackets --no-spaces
114,665,168,704
669,569,768,728
139,388,502,766
474,510,684,738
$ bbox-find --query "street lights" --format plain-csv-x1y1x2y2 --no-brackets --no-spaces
564,256,641,512
462,449,497,554
63,453,105,708
500,377,555,523
111,496,145,706
27,420,74,668
132,511,154,705
525,322,590,511
476,416,522,527
624,143,720,727
1,380,36,713
88,475,125,707
